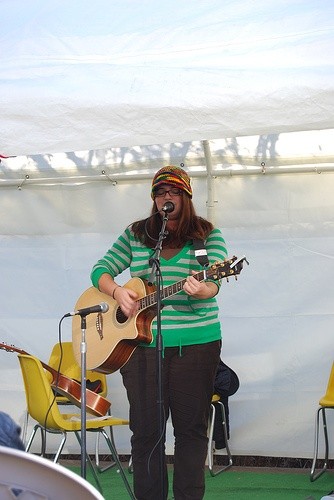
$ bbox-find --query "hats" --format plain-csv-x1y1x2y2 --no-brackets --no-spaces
151,166,192,200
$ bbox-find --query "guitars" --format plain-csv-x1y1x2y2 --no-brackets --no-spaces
72,255,246,375
0,343,111,416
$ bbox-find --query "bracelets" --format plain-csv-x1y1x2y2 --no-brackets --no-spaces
113,286,121,300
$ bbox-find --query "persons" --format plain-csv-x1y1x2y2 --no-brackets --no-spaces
0,411,26,496
212,359,239,439
91,166,227,500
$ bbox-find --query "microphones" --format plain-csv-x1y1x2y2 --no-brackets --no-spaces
162,202,174,212
64,302,109,317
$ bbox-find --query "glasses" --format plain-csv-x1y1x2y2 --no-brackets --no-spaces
154,188,184,197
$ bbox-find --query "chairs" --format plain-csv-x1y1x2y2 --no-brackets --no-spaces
127,394,233,478
309,362,334,481
18,354,136,500
18,341,118,472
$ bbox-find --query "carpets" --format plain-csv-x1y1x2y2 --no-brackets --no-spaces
63,466,334,500
